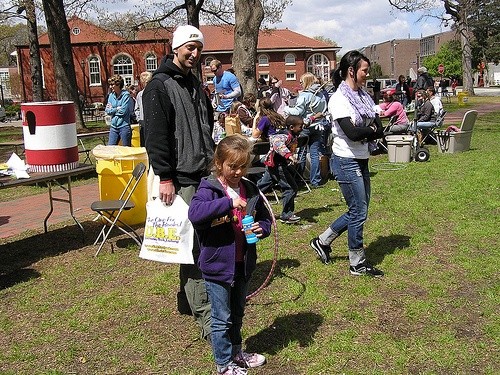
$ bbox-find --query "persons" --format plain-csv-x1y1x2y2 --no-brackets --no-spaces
105,72,153,147
309,50,385,278
187,136,273,375
377,65,458,148
204,59,342,223
142,25,217,345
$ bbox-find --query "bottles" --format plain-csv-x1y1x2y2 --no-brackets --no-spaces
241,214,258,243
213,125,218,142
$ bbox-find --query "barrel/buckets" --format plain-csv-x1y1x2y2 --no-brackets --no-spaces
21,101,80,173
118,124,141,147
93,144,149,227
457,92,469,106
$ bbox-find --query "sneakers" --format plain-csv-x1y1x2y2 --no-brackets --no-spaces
350,262,384,277
309,236,332,264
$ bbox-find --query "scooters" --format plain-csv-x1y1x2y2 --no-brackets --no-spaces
368,109,448,162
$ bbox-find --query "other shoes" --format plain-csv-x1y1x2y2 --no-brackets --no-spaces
234,352,266,368
279,214,301,224
216,363,250,375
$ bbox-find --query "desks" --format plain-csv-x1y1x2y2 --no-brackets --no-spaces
2,141,25,160
0,161,95,244
76,131,110,164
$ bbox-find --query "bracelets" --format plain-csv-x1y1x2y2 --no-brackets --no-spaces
159,180,175,184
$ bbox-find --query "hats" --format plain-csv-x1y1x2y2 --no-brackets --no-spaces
171,25,204,50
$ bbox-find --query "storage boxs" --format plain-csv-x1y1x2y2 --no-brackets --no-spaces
435,110,480,154
386,134,415,164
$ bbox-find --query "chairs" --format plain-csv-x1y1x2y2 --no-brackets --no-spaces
90,163,142,256
368,115,396,155
290,136,313,192
243,139,281,205
418,111,445,146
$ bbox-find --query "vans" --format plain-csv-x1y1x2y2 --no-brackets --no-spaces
361,78,398,96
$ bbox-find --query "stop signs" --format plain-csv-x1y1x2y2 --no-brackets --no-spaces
438,64,444,74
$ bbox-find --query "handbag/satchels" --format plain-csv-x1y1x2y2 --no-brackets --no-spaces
138,196,196,265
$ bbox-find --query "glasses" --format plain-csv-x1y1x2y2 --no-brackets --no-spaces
417,95,422,98
109,83,115,86
211,67,219,72
